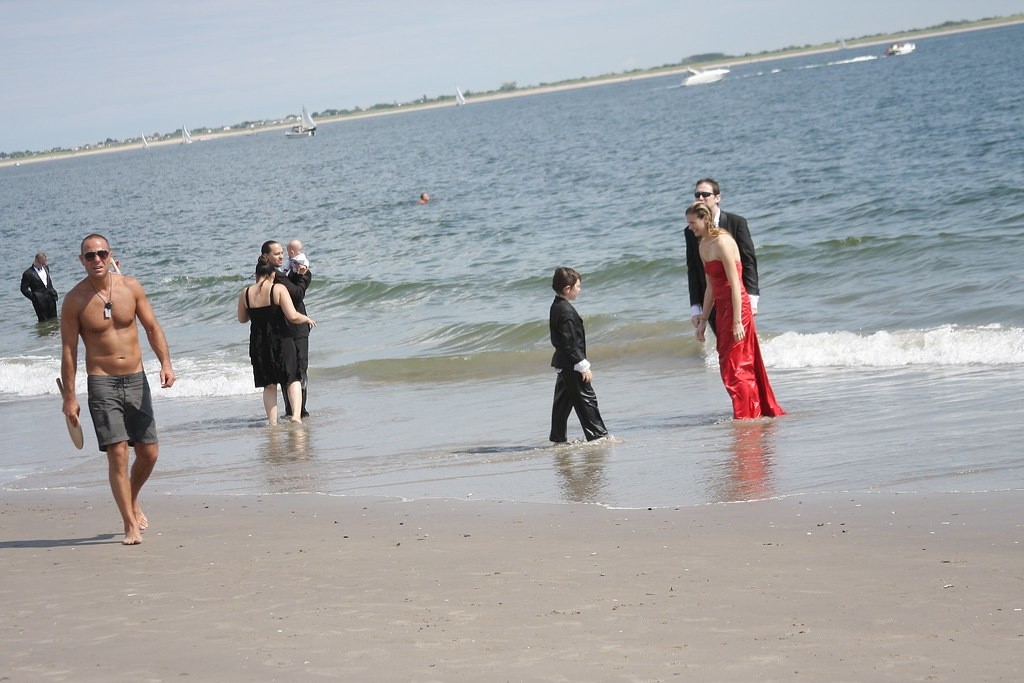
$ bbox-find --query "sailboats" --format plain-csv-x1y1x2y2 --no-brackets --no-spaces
180,124,193,145
284,103,318,139
454,85,466,109
139,131,150,150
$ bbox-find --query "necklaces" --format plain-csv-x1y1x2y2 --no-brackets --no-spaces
88,272,113,317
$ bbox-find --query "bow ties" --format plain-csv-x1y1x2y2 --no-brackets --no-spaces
38,268,42,270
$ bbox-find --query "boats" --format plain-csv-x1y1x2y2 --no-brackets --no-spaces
679,65,730,88
882,41,914,57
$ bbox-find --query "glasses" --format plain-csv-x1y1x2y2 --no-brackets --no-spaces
84,249,109,262
694,192,715,198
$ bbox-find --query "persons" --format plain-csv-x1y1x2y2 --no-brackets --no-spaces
419,193,428,204
682,178,760,335
21,234,318,544
684,201,786,422
549,267,609,445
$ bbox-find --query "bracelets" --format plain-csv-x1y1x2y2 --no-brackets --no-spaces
700,318,709,321
582,369,589,373
